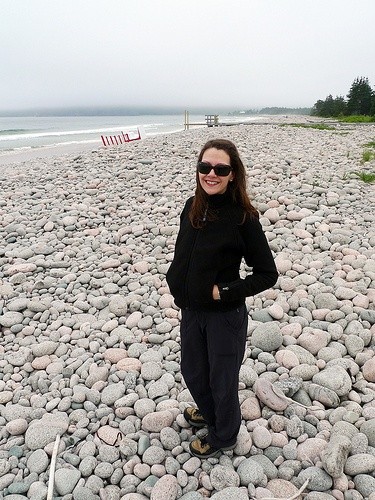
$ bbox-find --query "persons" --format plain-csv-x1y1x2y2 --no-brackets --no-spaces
165,139,278,460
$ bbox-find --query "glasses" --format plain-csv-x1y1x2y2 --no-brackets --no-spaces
197,162,234,176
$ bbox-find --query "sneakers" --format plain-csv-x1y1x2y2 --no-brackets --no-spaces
188,433,237,458
184,406,209,427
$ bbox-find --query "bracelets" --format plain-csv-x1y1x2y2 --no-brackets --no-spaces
218,289,221,301
